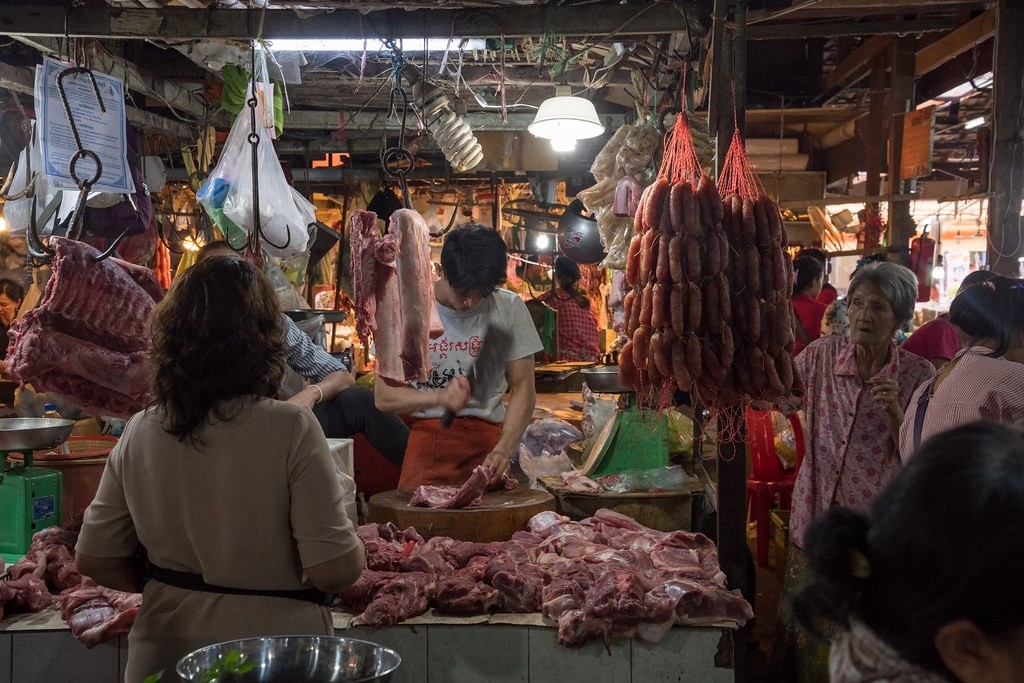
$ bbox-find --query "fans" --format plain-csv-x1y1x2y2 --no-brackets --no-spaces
807,207,865,252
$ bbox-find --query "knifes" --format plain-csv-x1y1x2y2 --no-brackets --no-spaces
442,326,514,426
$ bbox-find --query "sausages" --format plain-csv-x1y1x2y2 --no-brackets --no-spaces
617,177,805,408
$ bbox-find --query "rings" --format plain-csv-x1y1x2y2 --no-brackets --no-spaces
883,391,887,395
501,473,508,477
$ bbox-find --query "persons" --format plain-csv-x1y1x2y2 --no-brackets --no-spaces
536,256,601,362
0,279,24,401
785,262,937,683
790,420,1024,683
74,256,365,683
748,405,804,509
790,257,830,356
797,249,1024,466
195,242,410,467
375,223,544,490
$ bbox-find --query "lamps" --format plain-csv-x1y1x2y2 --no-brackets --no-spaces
528,85,606,151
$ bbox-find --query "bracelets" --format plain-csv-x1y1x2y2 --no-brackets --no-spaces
307,385,322,404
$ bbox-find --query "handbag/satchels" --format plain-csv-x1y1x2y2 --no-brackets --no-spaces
196,82,318,262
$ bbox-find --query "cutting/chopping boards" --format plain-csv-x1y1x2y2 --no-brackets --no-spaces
368,485,556,543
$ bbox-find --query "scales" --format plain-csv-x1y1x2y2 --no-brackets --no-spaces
579,366,672,481
0,416,75,570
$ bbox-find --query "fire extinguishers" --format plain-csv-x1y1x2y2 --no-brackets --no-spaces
910,223,937,302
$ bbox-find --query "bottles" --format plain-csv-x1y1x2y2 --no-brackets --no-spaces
42,404,61,419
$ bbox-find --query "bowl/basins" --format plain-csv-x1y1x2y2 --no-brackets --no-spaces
176,634,403,683
